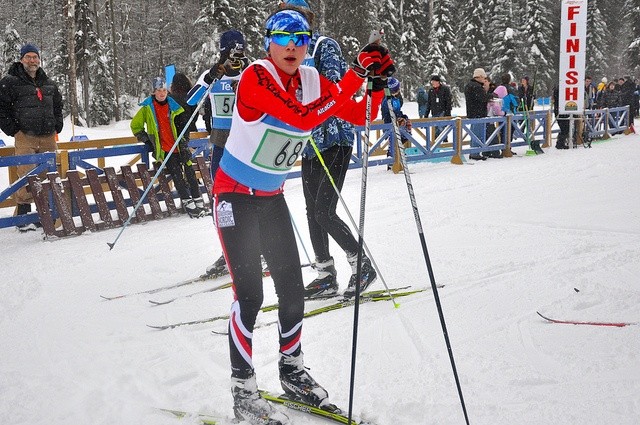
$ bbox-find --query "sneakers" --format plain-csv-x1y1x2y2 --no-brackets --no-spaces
555,143,568,148
489,152,504,158
182,199,202,216
193,197,210,213
469,154,482,159
207,252,227,275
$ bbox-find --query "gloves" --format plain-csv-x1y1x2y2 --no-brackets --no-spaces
407,119,412,130
350,41,383,76
144,139,154,152
229,53,251,71
373,49,396,79
204,63,227,84
397,116,406,127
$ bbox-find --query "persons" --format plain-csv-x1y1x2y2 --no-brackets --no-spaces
272,0,377,300
0,44,64,232
382,77,411,170
211,9,396,424
552,76,640,149
417,86,429,129
185,30,258,276
131,76,209,218
424,76,452,142
168,73,201,184
465,68,536,160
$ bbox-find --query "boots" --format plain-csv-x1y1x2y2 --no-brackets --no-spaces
344,249,376,298
231,370,289,425
303,257,338,298
277,350,328,406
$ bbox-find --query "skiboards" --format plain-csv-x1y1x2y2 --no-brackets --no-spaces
537,303,635,339
99,268,312,306
145,286,446,336
161,389,364,425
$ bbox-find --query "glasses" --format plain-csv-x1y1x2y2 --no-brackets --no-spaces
265,30,312,46
23,55,39,60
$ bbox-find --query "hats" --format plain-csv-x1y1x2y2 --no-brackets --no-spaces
220,30,245,51
284,0,310,11
473,67,487,78
153,76,167,90
262,10,313,53
432,75,440,81
20,44,39,58
387,76,400,91
